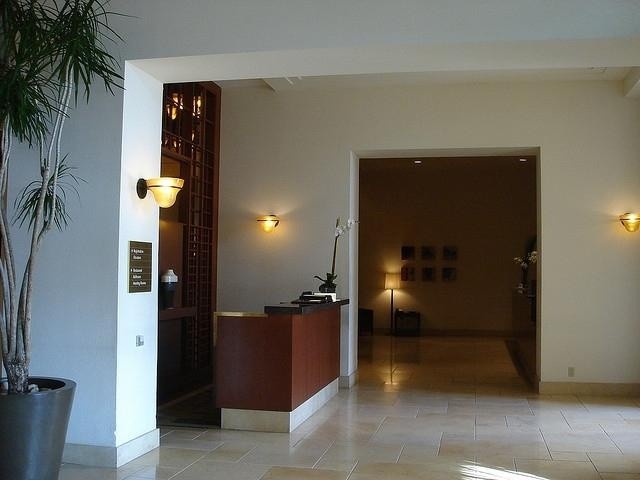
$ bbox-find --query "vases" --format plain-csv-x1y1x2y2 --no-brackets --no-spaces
318,283,336,294
521,262,529,288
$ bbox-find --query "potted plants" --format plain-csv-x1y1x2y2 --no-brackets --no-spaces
0,1,150,480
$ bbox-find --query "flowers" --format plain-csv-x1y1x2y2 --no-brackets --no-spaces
510,249,537,267
314,217,362,283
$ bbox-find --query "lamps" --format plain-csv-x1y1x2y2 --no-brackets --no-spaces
255,212,281,232
620,212,640,234
136,177,184,208
384,271,401,337
160,267,180,310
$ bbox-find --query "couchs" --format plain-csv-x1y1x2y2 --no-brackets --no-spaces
358,308,374,336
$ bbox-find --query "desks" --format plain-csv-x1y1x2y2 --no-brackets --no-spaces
394,309,420,336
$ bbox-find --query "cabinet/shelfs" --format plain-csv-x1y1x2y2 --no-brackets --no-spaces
511,290,536,377
